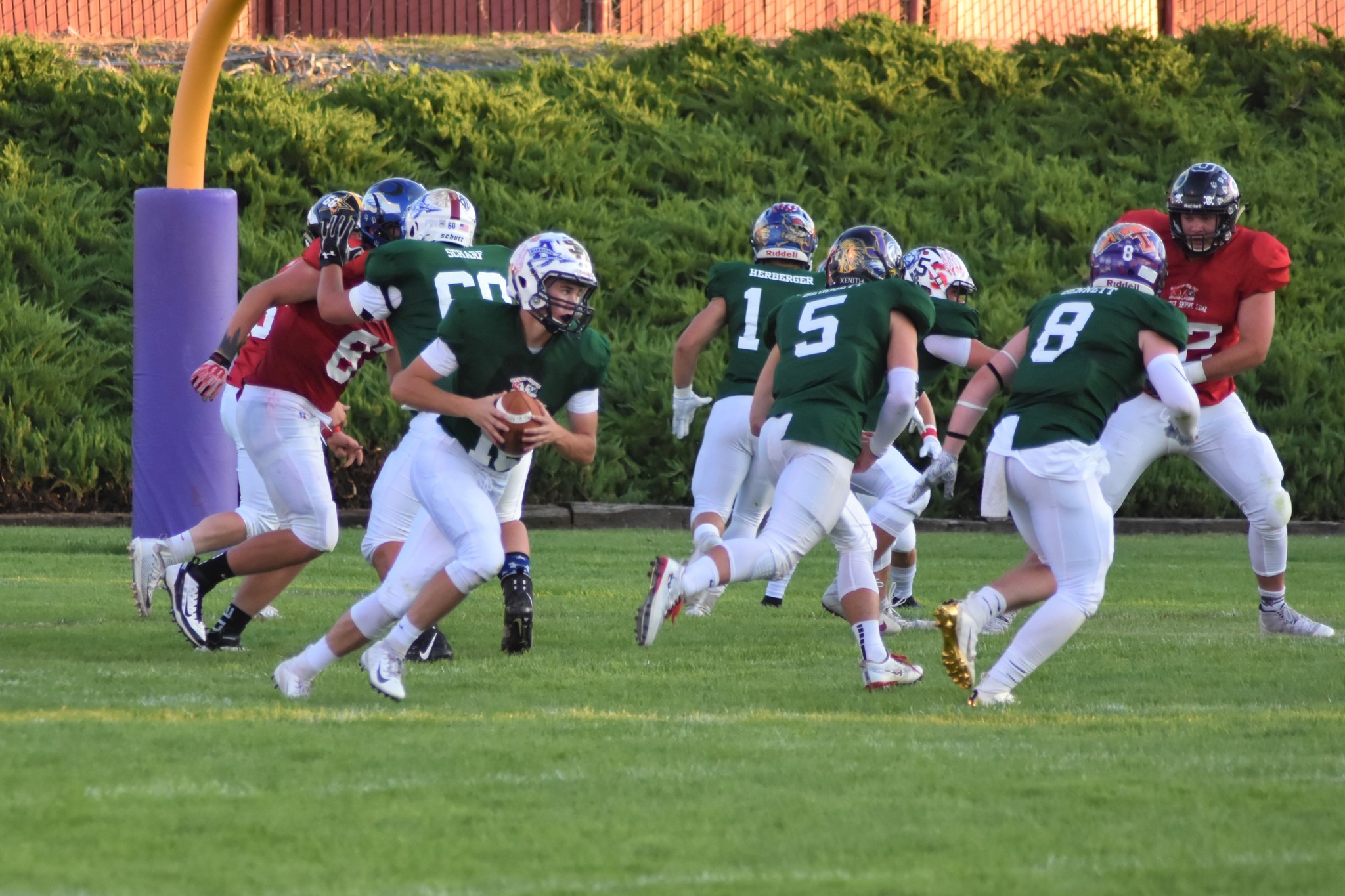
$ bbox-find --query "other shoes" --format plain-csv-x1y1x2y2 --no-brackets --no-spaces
684,584,730,618
685,535,723,566
893,594,921,607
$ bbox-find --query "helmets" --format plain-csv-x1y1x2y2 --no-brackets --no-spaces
750,202,817,262
826,225,907,286
360,177,427,248
1088,223,1167,295
1167,163,1239,255
507,232,598,322
404,188,478,248
306,191,363,247
902,247,975,300
816,259,826,272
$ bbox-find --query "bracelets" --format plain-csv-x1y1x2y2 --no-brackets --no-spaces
321,426,341,441
946,430,969,441
921,428,938,440
210,352,231,371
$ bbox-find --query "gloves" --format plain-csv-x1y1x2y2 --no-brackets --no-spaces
906,452,959,504
318,214,367,267
919,424,943,460
1163,419,1197,446
908,407,926,435
672,383,713,440
191,351,231,403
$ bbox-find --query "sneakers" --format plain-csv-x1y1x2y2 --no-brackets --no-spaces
405,626,453,662
232,593,283,620
967,672,1020,704
1258,602,1334,638
879,581,910,630
158,556,207,649
193,627,248,652
821,578,900,636
981,608,1019,635
858,650,925,690
498,566,535,655
357,638,405,701
633,555,684,647
269,655,321,701
126,532,173,618
933,599,978,688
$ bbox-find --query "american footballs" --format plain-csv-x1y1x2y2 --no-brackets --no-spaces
493,389,542,458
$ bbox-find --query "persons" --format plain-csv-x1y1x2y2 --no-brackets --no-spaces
907,222,1201,709
672,203,824,617
126,180,426,654
635,227,934,689
1101,163,1337,639
823,246,1002,634
273,233,608,705
318,188,533,659
